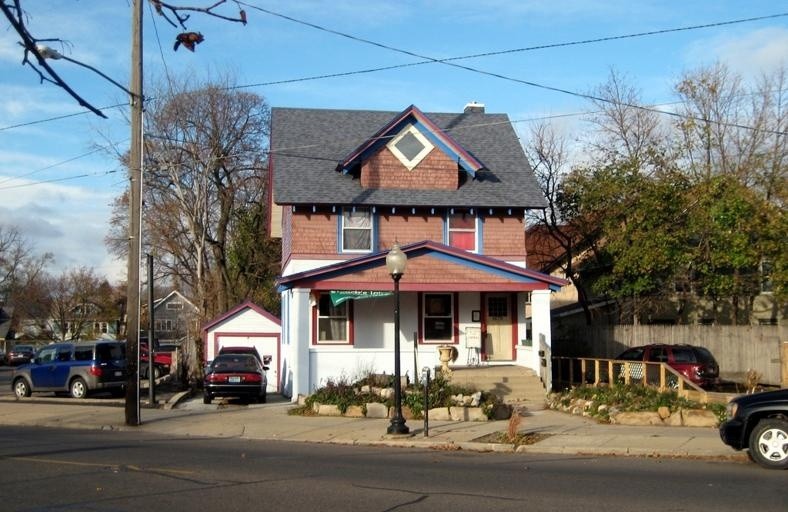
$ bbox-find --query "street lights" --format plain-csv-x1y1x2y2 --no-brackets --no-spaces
386,245,407,434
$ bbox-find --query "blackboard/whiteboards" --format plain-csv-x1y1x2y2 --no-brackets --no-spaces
465,328,481,349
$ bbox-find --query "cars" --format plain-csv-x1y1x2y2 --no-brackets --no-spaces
6,340,181,397
203,347,268,402
614,344,719,390
719,389,788,469
